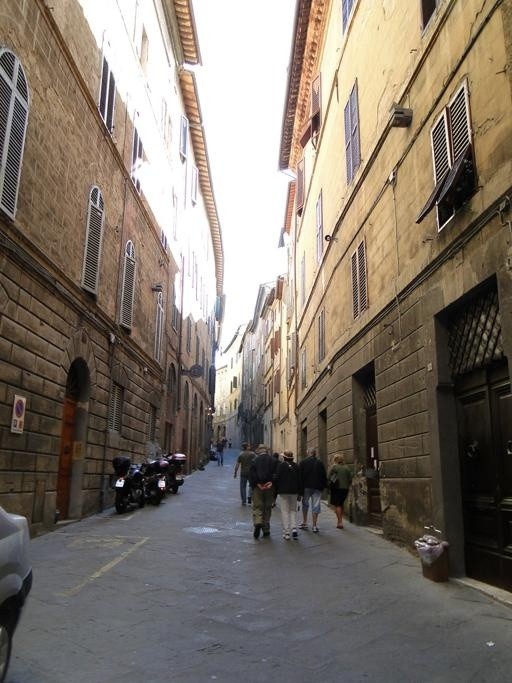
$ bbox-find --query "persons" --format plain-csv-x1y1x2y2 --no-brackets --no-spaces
217,438,224,467
234,442,352,541
229,438,232,448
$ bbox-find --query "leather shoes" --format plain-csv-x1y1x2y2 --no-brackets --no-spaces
264,532,270,536
253,523,262,539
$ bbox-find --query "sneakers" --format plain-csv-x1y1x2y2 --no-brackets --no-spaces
282,532,291,540
298,522,309,529
312,526,320,532
292,529,298,537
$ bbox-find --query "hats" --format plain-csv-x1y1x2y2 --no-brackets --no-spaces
307,448,317,457
281,449,295,459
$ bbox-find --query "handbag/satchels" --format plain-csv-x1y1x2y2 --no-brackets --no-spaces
328,469,339,489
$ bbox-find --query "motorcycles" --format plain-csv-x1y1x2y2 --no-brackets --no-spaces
110,449,186,513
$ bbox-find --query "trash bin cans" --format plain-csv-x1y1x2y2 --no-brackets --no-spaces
414,534,450,582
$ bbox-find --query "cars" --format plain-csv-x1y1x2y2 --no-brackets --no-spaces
1,497,37,683
